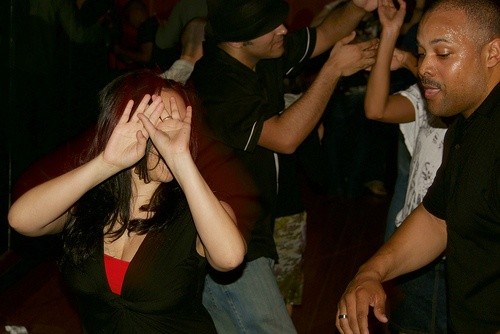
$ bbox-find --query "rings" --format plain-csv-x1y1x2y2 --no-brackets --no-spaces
339,315,348,319
162,116,172,122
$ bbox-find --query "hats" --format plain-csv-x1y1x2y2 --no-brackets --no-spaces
206,0,289,42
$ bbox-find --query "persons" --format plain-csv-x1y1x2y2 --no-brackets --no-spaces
7,69,247,334
109,0,452,334
336,1,500,334
